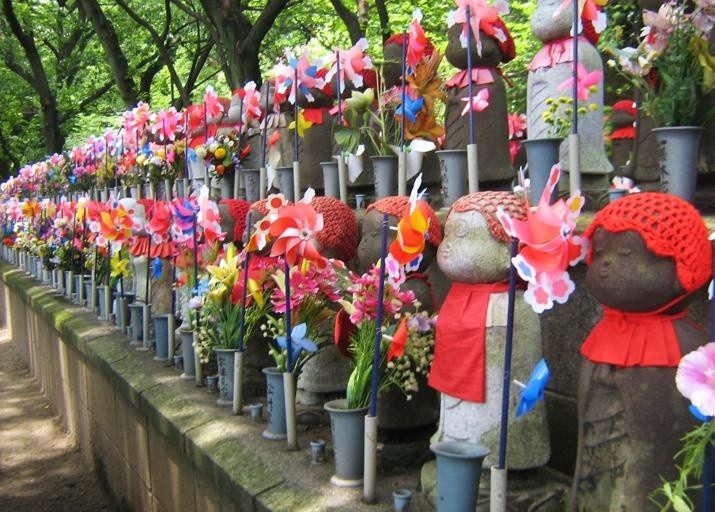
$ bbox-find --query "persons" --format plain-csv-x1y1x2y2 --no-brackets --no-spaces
629,86,713,213
434,7,516,196
188,34,441,196
513,1,614,210
568,193,713,512
428,189,550,494
206,196,360,423
350,195,442,457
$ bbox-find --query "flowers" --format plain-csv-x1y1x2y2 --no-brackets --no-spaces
1,0,714,511
